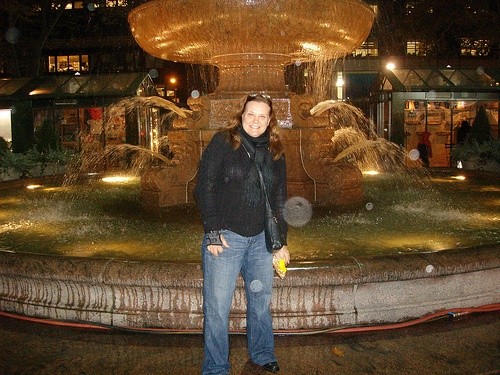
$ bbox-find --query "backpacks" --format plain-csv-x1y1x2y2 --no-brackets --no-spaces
418,142,429,160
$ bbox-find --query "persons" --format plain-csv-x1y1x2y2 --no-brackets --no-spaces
417,133,432,167
195,92,290,375
456,121,471,143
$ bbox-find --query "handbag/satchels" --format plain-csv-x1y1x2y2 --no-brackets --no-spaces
265,206,288,252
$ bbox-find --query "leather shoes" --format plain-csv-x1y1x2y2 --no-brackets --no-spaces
262,361,279,372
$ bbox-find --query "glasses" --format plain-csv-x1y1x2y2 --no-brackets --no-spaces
248,93,272,100
